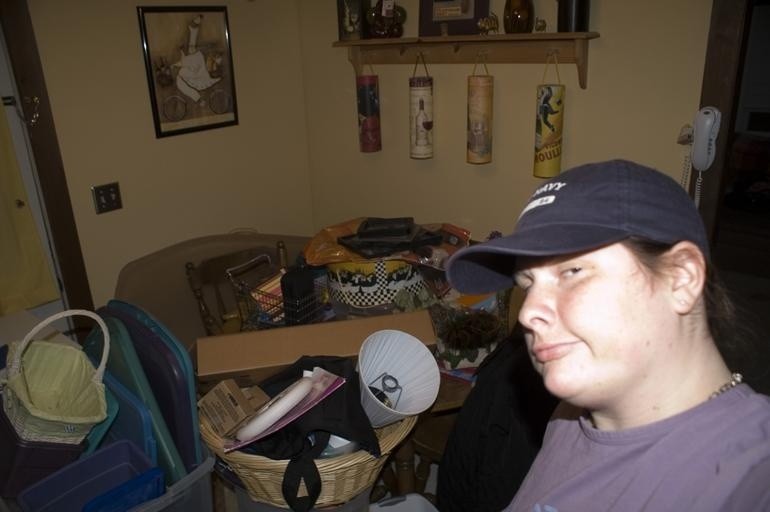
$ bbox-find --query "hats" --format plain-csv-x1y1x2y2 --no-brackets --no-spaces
445,159,714,296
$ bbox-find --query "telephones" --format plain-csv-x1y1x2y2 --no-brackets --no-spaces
691,106,721,171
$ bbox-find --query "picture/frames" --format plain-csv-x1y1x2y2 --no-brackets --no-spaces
136,4,240,139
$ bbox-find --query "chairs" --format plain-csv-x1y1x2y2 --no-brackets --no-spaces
185,239,287,336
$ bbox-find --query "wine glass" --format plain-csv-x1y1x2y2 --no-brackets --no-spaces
422,119,433,147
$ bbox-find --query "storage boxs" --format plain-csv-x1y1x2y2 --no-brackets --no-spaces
183,301,438,424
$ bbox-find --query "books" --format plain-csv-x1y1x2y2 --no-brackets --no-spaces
222,365,346,456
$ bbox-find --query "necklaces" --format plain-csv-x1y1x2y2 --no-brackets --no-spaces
709,371,744,402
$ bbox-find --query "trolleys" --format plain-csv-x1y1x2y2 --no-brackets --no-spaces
225,253,328,332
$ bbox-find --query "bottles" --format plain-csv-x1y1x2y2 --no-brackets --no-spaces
415,98,431,147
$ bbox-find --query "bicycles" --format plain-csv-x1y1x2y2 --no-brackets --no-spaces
161,65,231,123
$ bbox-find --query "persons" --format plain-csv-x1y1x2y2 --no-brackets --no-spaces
441,156,768,511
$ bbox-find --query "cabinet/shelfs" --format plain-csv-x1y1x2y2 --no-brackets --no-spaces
331,0,600,179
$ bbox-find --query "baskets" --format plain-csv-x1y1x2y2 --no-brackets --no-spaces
0,309,112,446
197,375,419,509
